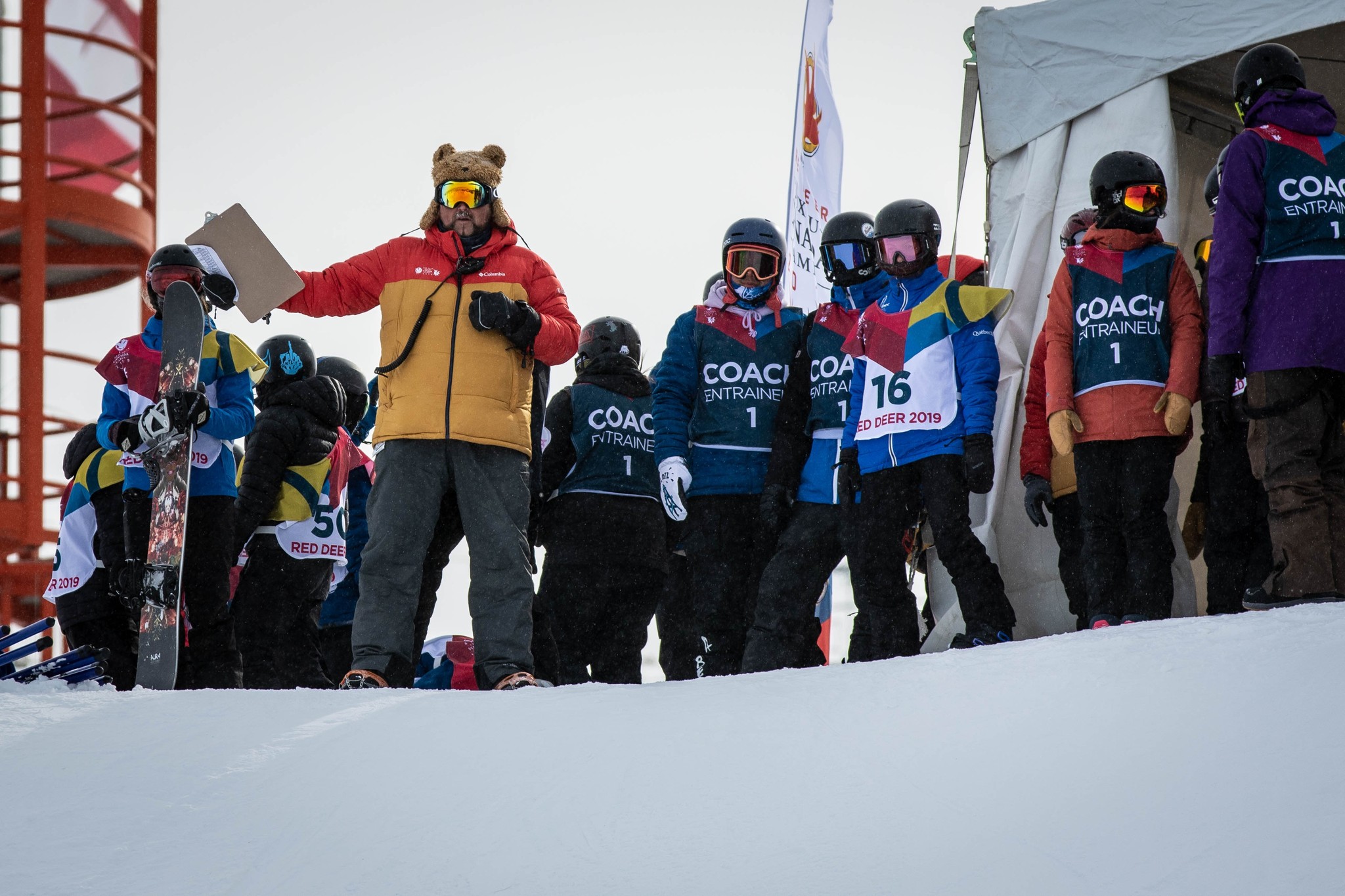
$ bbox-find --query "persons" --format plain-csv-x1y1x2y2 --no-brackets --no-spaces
201,142,581,693
541,315,691,686
179,444,247,689
349,359,562,687
93,245,269,690
651,218,812,677
1180,140,1274,615
1045,150,1204,629
739,212,919,678
314,353,378,690
229,335,363,691
650,270,826,682
834,198,1017,665
1018,208,1104,631
1205,42,1344,611
902,255,990,647
43,423,138,697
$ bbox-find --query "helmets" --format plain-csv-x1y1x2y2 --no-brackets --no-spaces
581,200,948,370
254,334,374,423
1061,42,1308,248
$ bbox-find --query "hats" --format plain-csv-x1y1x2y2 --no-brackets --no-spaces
420,143,511,230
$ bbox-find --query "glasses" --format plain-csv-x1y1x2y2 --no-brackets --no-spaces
726,247,779,280
821,240,878,273
1123,183,1170,214
876,233,933,267
434,181,495,210
153,265,204,298
1069,229,1089,246
1196,239,1212,271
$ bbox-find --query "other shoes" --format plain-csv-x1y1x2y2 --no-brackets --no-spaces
494,671,531,691
952,624,1013,648
1088,613,1121,629
340,668,383,689
1239,583,1335,611
1120,614,1151,626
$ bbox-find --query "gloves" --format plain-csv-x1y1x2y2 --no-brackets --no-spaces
1207,354,1251,409
963,409,1084,528
1153,390,1192,435
831,445,867,512
138,389,212,448
658,453,691,521
199,273,236,311
468,289,529,337
758,482,794,531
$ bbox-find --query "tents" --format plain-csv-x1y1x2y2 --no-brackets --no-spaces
917,0,1345,661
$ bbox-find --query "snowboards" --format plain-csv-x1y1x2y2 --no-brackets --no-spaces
133,280,206,690
